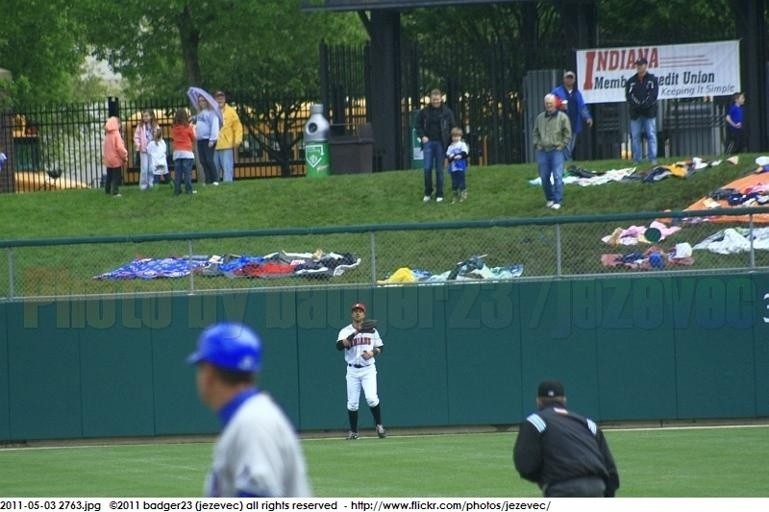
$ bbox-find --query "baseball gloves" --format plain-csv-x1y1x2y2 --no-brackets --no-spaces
358,321,378,333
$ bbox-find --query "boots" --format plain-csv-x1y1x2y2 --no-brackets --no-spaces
450,190,458,204
459,188,468,203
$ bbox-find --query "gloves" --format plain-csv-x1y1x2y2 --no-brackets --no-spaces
362,349,377,359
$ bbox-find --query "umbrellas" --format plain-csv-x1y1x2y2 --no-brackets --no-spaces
187,87,225,128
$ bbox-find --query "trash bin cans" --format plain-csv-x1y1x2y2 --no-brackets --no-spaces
327,122,375,173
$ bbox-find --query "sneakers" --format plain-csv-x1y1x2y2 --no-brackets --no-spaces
346,431,360,441
436,195,443,202
545,199,563,210
112,192,123,198
212,181,219,186
191,190,198,194
423,188,434,203
375,423,385,438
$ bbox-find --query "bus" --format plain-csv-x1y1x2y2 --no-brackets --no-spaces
10,113,90,193
118,86,524,185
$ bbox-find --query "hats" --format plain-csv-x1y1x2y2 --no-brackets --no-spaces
563,71,576,80
351,303,367,313
537,381,565,397
634,57,647,65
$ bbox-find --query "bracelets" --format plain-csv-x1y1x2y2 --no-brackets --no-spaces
372,349,379,355
347,332,355,341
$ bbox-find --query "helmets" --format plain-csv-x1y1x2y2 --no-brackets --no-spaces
182,322,263,376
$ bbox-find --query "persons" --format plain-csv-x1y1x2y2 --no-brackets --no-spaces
727,91,746,156
513,378,621,497
550,71,592,156
103,91,243,198
531,93,572,210
625,58,658,163
445,126,470,204
415,88,456,202
182,318,313,497
335,304,386,439
